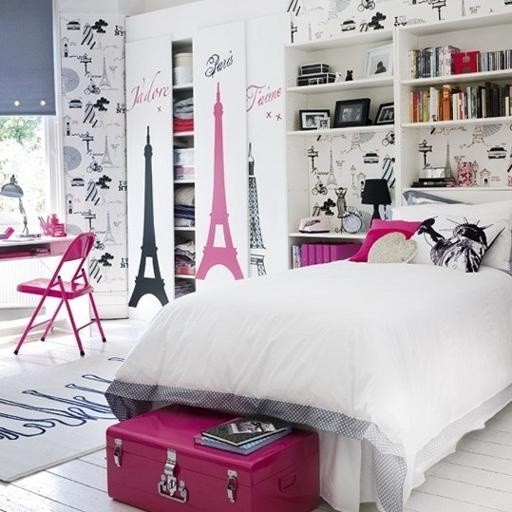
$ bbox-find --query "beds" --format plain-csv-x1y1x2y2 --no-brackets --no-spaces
104,188,511,512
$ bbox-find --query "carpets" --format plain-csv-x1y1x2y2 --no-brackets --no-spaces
0,353,128,484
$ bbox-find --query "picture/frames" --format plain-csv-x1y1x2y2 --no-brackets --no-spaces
333,98,370,128
374,103,394,125
300,110,330,130
365,44,393,79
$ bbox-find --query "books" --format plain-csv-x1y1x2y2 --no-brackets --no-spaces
191,416,294,458
407,44,512,123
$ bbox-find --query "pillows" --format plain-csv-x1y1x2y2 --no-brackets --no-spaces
349,192,511,273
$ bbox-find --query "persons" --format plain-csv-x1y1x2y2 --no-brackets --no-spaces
321,120,328,127
374,61,385,74
306,116,320,127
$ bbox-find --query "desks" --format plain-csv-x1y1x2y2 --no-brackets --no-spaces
0,237,91,337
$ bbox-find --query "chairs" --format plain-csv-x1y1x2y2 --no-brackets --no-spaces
13,232,106,356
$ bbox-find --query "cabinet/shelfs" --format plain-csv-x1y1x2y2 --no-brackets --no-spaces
124,0,310,320
283,28,396,240
396,10,512,189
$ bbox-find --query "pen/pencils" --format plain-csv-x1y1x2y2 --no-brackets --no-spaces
39,216,49,224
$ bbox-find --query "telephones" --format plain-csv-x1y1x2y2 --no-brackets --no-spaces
298,216,330,233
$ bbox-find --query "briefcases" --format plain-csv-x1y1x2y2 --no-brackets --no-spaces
106,404,320,511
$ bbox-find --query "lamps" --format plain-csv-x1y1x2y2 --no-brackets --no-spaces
361,179,391,228
0,175,36,238
